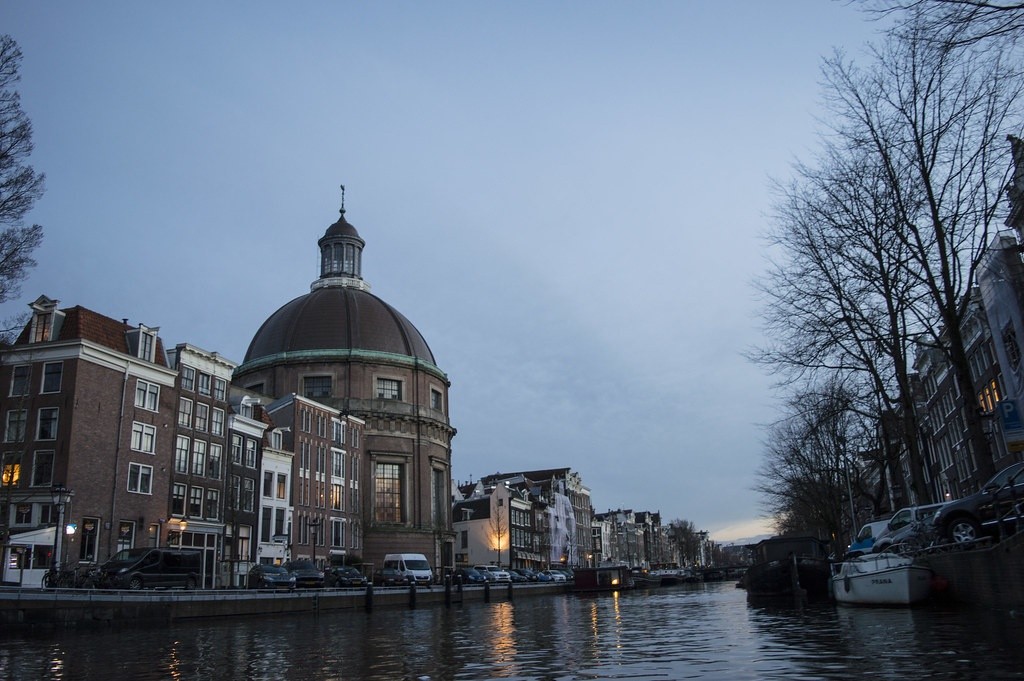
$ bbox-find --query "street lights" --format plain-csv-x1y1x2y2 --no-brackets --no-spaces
48,486,72,570
309,518,321,565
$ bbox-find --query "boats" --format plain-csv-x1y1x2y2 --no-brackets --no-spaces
568,563,704,593
741,534,831,596
829,540,935,606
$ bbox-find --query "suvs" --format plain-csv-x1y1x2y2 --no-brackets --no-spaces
282,559,326,591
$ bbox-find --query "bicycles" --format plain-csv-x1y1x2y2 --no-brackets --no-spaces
40,559,104,592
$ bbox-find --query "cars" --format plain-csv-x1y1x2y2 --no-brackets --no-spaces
327,564,372,590
367,568,408,586
454,563,575,584
848,461,1024,559
247,563,296,592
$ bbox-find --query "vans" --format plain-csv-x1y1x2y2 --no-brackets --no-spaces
97,545,204,593
382,551,434,586
